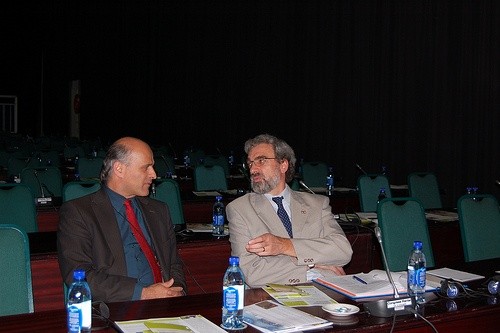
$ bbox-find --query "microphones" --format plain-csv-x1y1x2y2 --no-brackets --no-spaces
363,226,428,318
356,163,367,174
34,170,52,203
299,181,315,194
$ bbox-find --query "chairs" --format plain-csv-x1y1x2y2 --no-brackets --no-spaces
0,131,500,317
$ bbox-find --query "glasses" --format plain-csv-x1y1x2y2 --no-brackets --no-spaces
243,157,276,170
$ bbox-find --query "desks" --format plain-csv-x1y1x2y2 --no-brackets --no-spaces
0,258,500,333
26,207,464,313
35,186,410,232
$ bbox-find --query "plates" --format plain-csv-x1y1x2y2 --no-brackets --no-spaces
322,304,359,316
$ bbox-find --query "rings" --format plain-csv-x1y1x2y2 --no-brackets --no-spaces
263,247,265,252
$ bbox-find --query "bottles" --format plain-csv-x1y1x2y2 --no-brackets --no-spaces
221,256,245,328
184,151,191,178
467,188,472,194
407,241,426,297
66,269,92,333
228,154,234,169
74,170,80,182
473,188,479,200
326,168,334,196
167,168,172,180
213,197,224,239
381,166,387,177
377,187,387,214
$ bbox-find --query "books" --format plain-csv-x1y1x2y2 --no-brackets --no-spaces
312,269,438,300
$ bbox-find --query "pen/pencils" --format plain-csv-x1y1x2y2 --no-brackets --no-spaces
354,276,367,284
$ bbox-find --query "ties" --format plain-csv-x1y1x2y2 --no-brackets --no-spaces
272,196,293,238
123,199,162,284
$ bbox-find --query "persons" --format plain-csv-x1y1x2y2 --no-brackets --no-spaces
226,135,353,289
57,137,189,302
